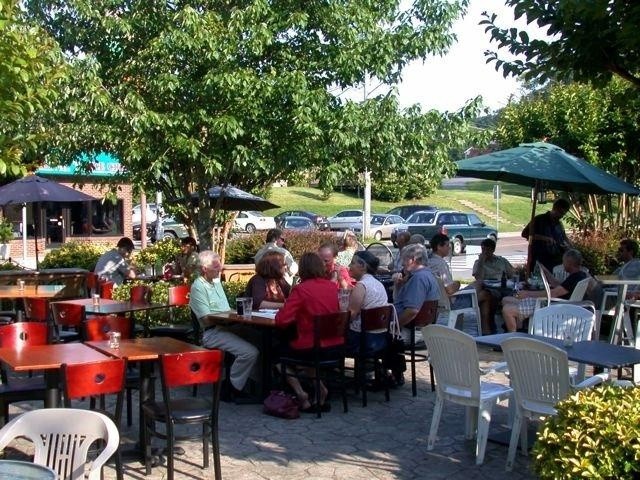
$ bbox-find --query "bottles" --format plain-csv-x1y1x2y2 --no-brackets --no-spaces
501,270,506,289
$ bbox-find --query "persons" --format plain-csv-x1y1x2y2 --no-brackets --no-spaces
93,200,640,413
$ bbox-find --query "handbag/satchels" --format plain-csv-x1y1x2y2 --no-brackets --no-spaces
263,382,302,420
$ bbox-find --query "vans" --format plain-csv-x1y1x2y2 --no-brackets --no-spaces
381,201,441,226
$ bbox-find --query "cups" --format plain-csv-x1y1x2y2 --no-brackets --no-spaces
512,275,520,290
92,293,100,305
108,331,122,348
19,281,25,291
236,297,253,318
337,286,351,309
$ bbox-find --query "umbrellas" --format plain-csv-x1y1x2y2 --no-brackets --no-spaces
443,136,640,282
0,170,97,265
166,186,281,212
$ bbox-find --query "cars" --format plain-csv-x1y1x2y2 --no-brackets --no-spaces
274,209,330,234
345,211,407,245
321,208,370,230
278,216,320,244
132,199,277,249
390,205,501,257
